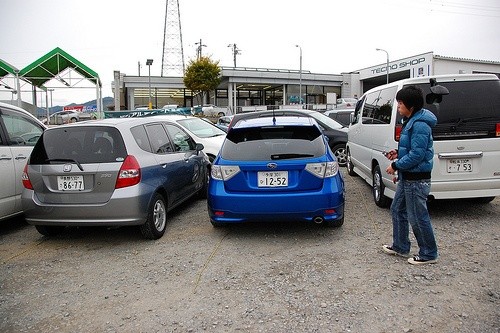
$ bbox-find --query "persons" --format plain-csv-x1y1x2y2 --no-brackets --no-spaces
382,85,438,265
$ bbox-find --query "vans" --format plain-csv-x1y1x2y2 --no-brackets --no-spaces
323,73,500,208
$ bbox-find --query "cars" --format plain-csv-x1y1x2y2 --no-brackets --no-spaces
0,102,233,241
290,95,303,103
217,110,345,229
336,97,357,109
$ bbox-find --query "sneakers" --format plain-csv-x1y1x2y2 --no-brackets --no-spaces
408,256,437,264
383,244,409,257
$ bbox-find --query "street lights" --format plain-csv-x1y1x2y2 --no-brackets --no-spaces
196,39,207,63
147,59,154,111
295,44,302,108
376,48,388,85
